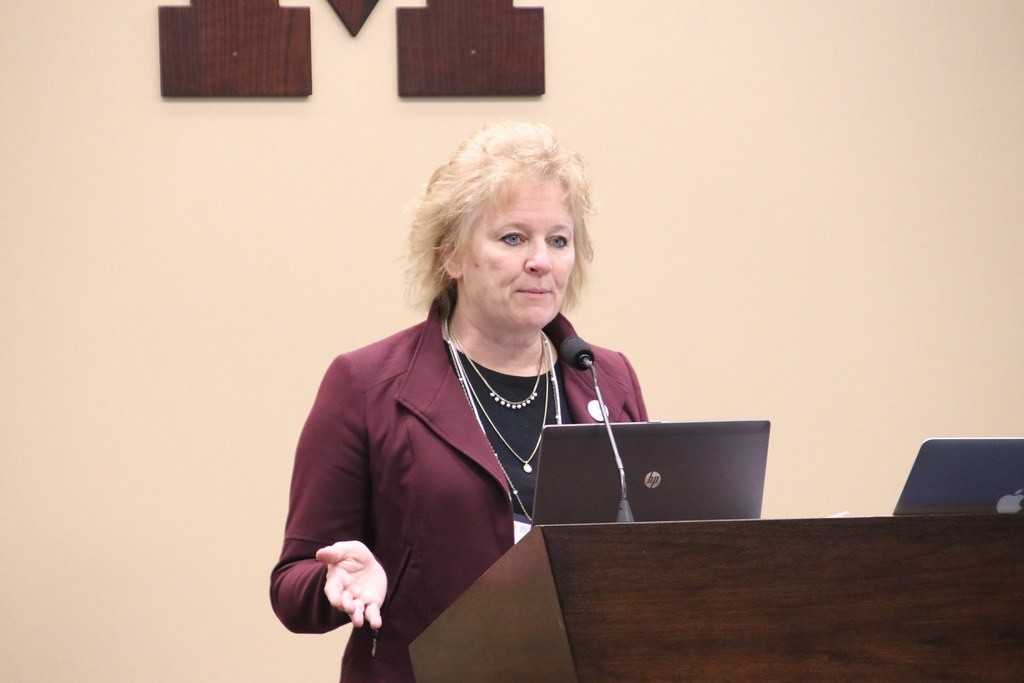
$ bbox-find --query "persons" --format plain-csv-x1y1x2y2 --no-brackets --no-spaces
269,120,649,683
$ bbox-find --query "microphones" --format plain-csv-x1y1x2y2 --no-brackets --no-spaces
560,335,637,523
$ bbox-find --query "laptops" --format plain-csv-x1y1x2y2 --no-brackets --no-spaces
530,421,771,530
893,437,1024,515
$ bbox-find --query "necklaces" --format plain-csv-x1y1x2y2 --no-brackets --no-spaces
443,313,563,525
